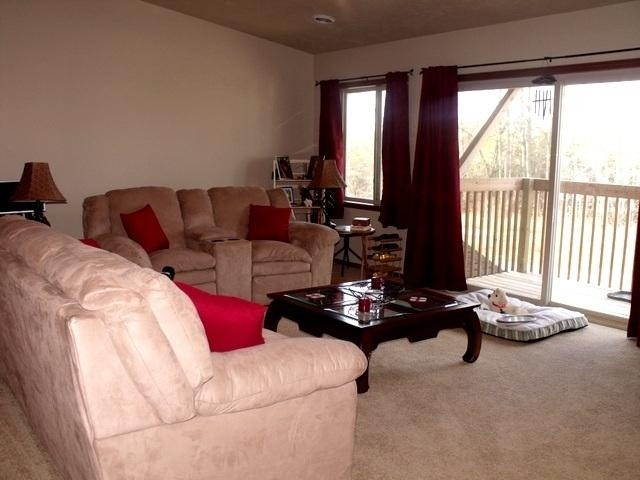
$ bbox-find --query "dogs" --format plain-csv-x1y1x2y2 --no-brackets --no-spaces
487,288,531,315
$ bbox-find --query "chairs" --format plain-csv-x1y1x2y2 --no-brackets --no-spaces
82,184,340,305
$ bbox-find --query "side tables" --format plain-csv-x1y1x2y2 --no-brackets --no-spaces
333,224,376,277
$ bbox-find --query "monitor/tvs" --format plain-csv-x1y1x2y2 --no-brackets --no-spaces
0,181,46,214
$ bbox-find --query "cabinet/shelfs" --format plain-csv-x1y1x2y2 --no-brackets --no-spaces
272,159,322,223
366,237,402,282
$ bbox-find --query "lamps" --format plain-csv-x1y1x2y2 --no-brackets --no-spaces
306,158,348,230
11,161,67,227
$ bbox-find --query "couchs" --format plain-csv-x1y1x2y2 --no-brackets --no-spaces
0,249,369,480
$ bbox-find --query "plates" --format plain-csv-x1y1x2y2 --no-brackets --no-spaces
497,315,537,323
306,293,325,300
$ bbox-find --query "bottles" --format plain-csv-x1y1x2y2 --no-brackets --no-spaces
359,297,369,312
371,277,380,289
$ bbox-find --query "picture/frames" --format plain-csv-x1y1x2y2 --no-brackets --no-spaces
281,187,294,204
